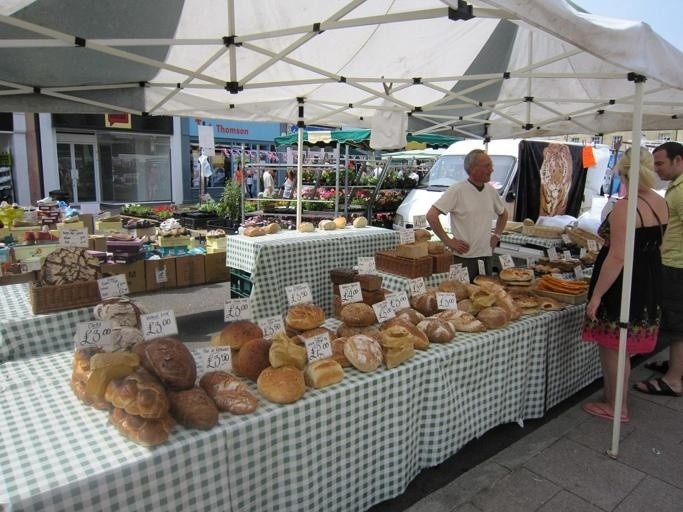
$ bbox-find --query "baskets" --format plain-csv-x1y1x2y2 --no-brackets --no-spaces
564,225,605,246
28,280,99,314
529,287,588,304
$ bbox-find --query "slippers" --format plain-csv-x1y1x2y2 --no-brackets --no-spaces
632,378,683,397
583,402,628,423
645,360,671,372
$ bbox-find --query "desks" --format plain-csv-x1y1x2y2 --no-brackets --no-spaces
498,232,580,258
226,233,402,325
544,274,619,413
1,314,551,510
1,279,142,366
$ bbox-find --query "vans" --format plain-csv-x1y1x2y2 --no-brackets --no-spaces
391,136,609,232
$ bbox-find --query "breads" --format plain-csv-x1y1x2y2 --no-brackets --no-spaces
426,239,446,255
413,228,432,243
245,215,369,237
565,224,606,251
215,252,598,404
391,241,430,260
505,222,524,233
523,218,562,238
199,367,257,416
71,296,218,447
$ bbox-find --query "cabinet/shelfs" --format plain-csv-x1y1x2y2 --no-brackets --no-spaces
241,145,438,224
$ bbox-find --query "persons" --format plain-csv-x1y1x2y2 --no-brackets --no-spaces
232,160,297,199
631,142,683,398
424,149,508,284
579,146,669,425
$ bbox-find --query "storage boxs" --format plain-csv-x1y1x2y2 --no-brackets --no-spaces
1,251,231,295
0,213,157,238
